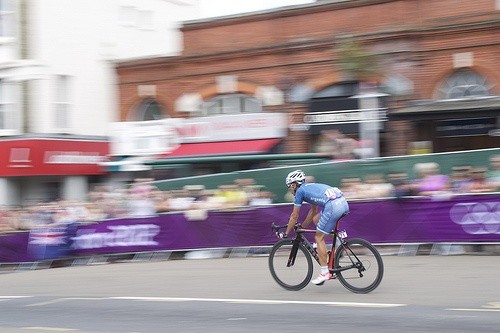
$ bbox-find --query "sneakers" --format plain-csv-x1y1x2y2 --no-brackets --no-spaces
330,273,336,279
312,272,330,285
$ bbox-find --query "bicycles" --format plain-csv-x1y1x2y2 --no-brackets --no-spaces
269,212,384,294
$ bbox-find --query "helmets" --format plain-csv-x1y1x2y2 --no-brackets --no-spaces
285,170,305,184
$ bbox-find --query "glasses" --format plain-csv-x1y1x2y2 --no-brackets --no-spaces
287,182,292,188
